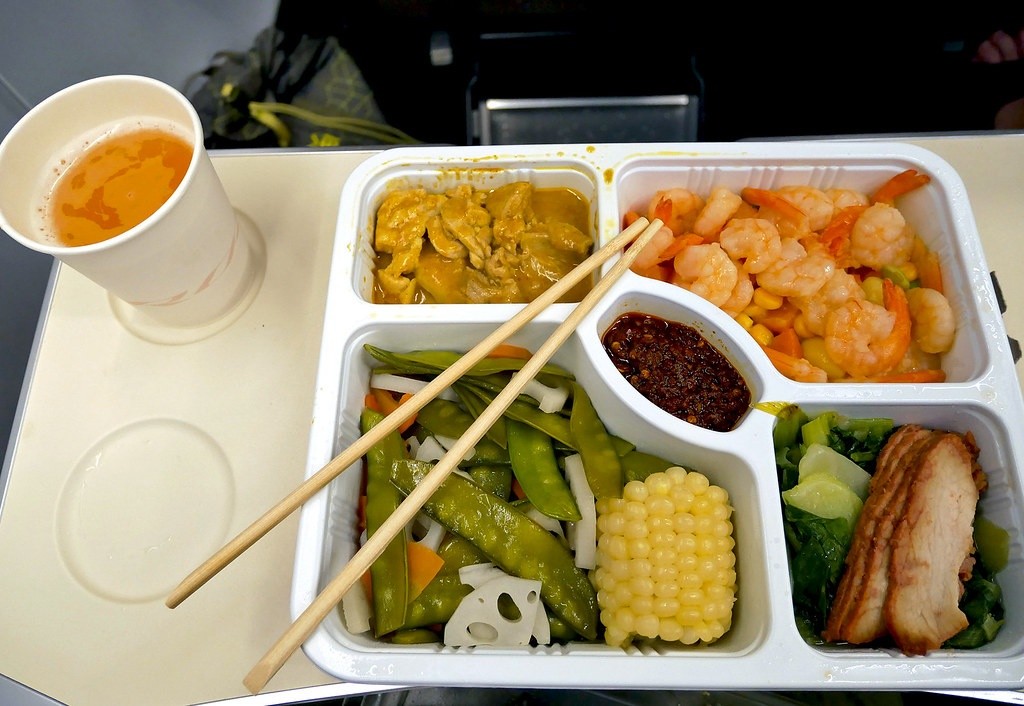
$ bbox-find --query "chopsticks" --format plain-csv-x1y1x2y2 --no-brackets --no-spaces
165,216,667,694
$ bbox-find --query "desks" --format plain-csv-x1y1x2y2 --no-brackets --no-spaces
0,131,1024,706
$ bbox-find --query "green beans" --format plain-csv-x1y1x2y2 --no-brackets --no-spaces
362,344,700,643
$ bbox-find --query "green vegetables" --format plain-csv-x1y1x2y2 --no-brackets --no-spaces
774,408,1013,652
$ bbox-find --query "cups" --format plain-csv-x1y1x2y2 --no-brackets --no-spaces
0,73,252,329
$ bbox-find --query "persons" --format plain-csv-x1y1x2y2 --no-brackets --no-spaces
277,0,1024,146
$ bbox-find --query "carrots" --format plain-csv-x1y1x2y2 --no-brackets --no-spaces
360,542,445,607
373,389,419,435
486,344,531,359
365,394,381,414
399,393,415,405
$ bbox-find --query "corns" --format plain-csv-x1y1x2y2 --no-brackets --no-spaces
588,466,737,646
